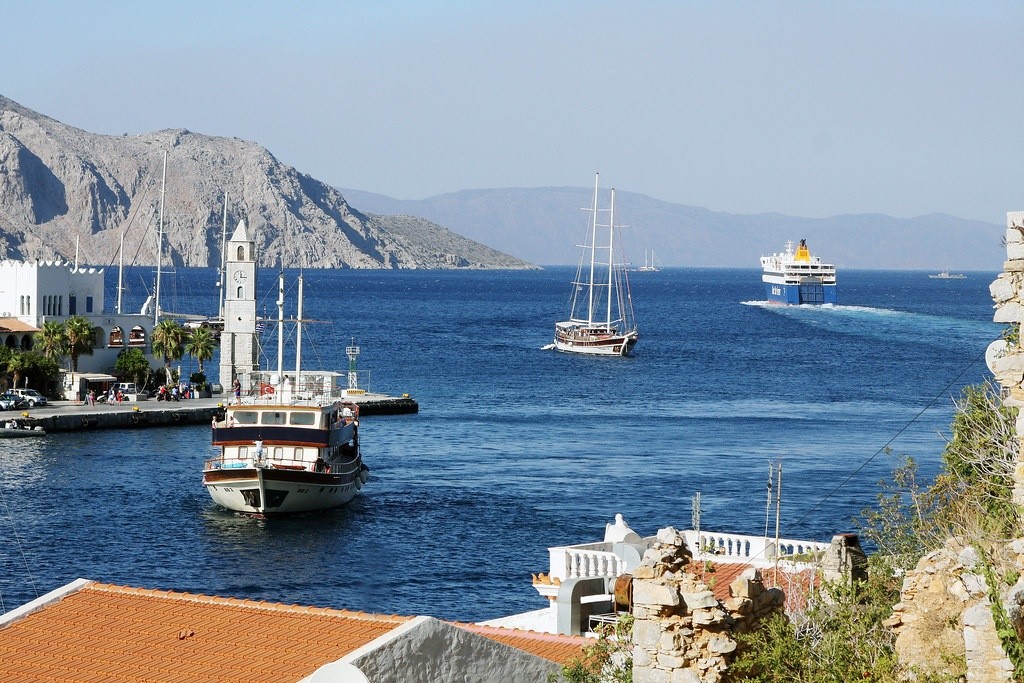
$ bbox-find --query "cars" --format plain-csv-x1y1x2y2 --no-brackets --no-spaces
109,382,135,394
0,394,30,411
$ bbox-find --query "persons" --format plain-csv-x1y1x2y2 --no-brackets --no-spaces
84,383,179,406
284,375,289,385
252,435,264,461
212,414,218,429
11,418,17,429
314,457,333,473
234,379,241,404
339,415,347,426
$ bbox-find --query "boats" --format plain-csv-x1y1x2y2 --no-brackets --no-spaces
200,256,370,519
760,239,838,307
928,267,968,279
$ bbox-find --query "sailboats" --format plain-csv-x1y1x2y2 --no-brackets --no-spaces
116,150,266,332
638,249,664,272
554,171,640,357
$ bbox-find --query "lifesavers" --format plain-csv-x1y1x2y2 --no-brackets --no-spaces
355,406,358,417
311,458,330,473
264,387,271,394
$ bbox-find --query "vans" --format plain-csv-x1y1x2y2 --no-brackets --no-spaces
8,388,48,407
